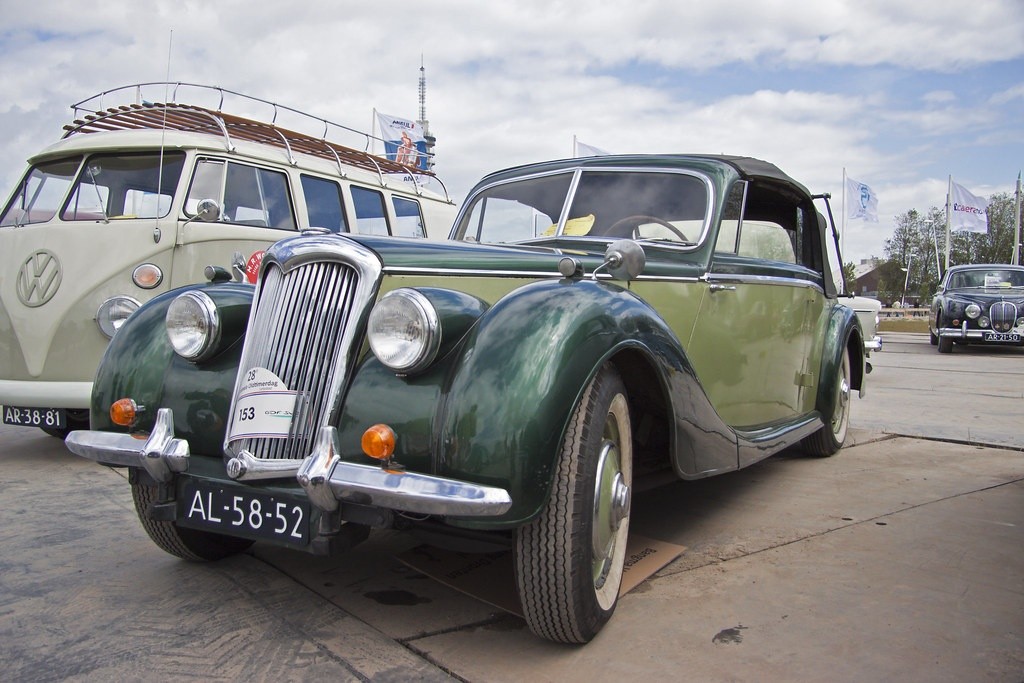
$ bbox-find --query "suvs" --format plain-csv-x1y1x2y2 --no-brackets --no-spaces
927,263,1024,354
62,152,870,647
811,191,883,357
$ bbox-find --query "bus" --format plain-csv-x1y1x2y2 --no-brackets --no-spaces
0,82,463,443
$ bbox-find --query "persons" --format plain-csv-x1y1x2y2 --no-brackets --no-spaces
913,299,920,317
886,299,892,317
922,299,928,316
892,299,900,317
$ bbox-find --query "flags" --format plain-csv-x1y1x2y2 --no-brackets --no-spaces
846,178,878,222
949,182,988,235
